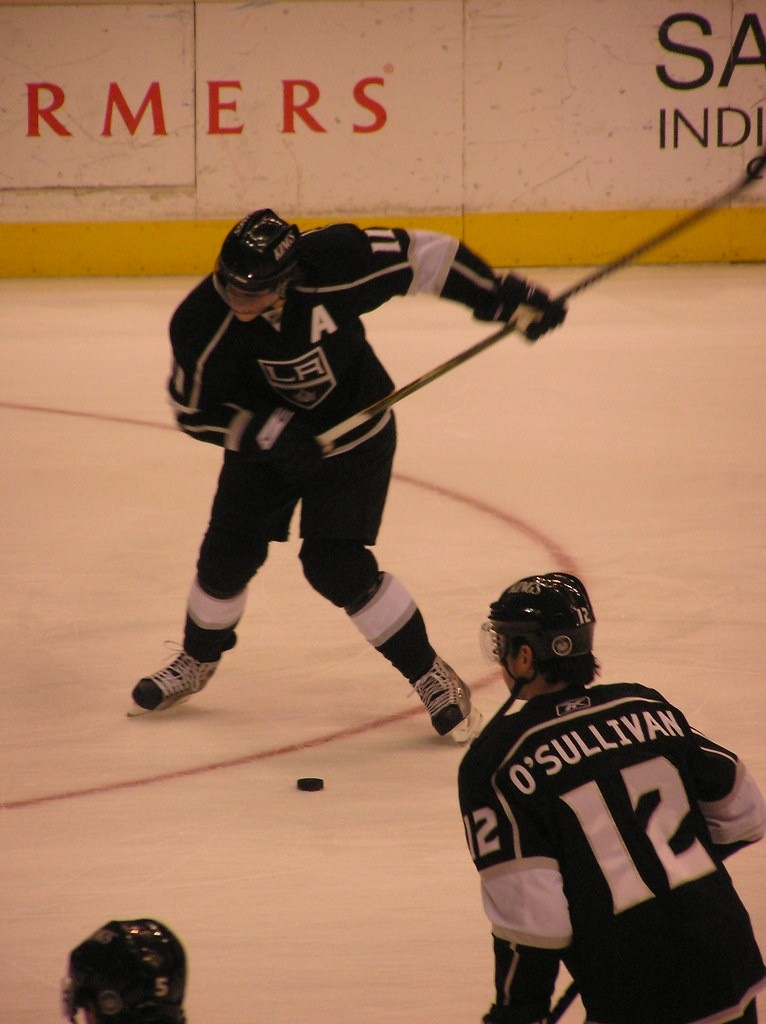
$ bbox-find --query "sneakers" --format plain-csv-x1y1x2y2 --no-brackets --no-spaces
126,640,222,718
406,656,481,743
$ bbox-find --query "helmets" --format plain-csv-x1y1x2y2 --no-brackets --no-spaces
480,573,595,668
59,918,185,1024
212,208,306,315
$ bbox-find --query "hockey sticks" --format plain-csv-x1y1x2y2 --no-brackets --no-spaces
314,151,766,464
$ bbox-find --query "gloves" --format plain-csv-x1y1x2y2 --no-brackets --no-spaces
238,404,332,479
472,273,568,343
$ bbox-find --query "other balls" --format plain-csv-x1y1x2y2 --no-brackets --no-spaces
297,776,324,792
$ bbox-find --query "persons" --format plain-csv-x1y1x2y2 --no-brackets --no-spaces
61,918,187,1024
131,209,568,744
458,572,766,1024
